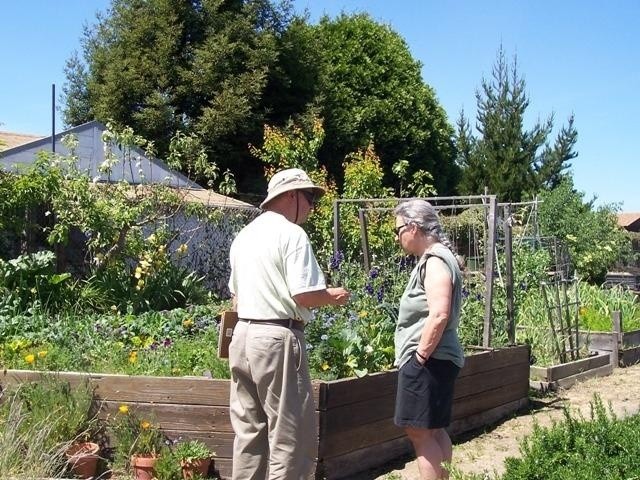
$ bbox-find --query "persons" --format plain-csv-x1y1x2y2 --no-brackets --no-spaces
392,199,466,480
227,167,351,480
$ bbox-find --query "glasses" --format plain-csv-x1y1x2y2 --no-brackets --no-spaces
392,223,410,237
297,188,316,206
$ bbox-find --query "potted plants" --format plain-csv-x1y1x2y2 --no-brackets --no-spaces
177,438,217,480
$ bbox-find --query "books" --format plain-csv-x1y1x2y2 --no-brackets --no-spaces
217,312,239,358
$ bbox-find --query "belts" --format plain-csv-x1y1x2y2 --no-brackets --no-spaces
239,316,304,332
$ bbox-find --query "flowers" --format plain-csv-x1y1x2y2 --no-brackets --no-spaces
130,404,172,456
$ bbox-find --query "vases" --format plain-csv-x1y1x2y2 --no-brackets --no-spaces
130,452,159,480
65,442,100,480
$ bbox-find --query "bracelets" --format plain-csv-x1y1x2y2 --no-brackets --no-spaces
416,351,428,361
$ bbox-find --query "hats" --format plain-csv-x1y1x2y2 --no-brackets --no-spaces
259,168,325,211
